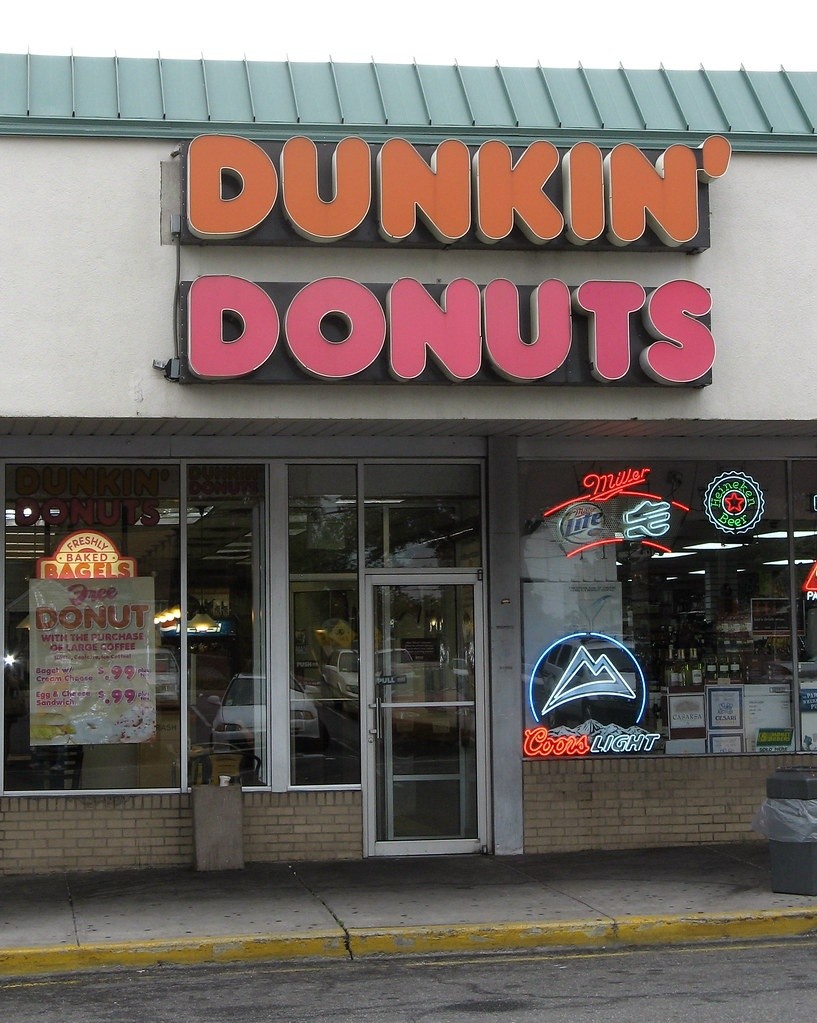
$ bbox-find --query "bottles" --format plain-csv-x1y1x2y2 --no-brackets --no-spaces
660,638,746,686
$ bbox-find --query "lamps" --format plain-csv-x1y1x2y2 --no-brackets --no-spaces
138,505,222,633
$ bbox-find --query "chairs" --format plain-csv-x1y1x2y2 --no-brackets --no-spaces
191,742,262,786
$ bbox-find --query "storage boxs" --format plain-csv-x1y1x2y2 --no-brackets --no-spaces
650,661,817,756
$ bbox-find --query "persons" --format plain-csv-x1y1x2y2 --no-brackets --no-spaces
196,695,222,783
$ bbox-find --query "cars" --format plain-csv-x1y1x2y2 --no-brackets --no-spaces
207,673,330,749
321,648,413,714
451,658,469,677
109,649,181,712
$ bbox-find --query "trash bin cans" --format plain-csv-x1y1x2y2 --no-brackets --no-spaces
759,763,817,896
